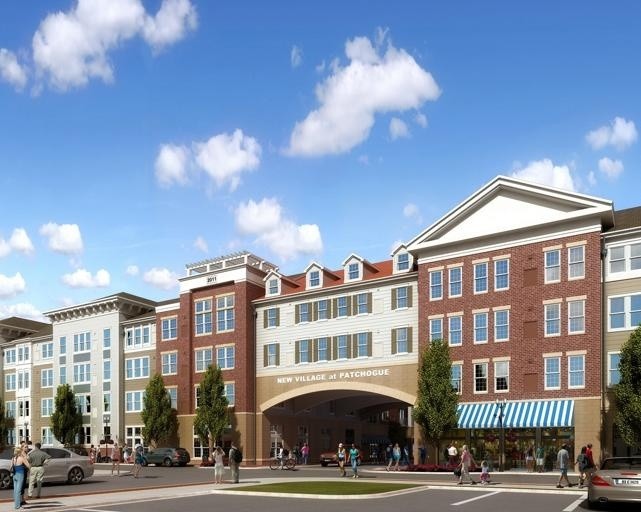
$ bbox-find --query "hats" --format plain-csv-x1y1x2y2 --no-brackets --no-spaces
338,442,344,448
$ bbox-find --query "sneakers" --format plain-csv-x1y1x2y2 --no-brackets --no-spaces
27,495,41,499
232,480,239,484
352,473,360,478
111,473,138,479
456,482,464,485
214,481,222,485
470,481,478,486
340,471,346,477
385,465,401,472
16,500,27,510
481,480,490,485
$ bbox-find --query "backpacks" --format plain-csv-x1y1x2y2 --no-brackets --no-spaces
231,447,243,464
135,451,142,465
282,448,289,456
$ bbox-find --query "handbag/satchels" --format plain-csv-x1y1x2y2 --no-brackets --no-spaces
454,466,461,477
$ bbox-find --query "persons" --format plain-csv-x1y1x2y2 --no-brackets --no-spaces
110,442,122,477
443,440,492,486
337,442,347,478
554,443,576,489
11,441,32,510
574,445,592,488
228,440,243,484
211,445,227,485
122,443,130,464
536,442,546,472
347,442,360,478
279,445,290,470
88,444,96,464
300,442,311,465
585,441,596,482
96,444,101,462
27,441,52,500
523,445,534,473
291,443,302,464
133,443,145,478
381,440,428,474
